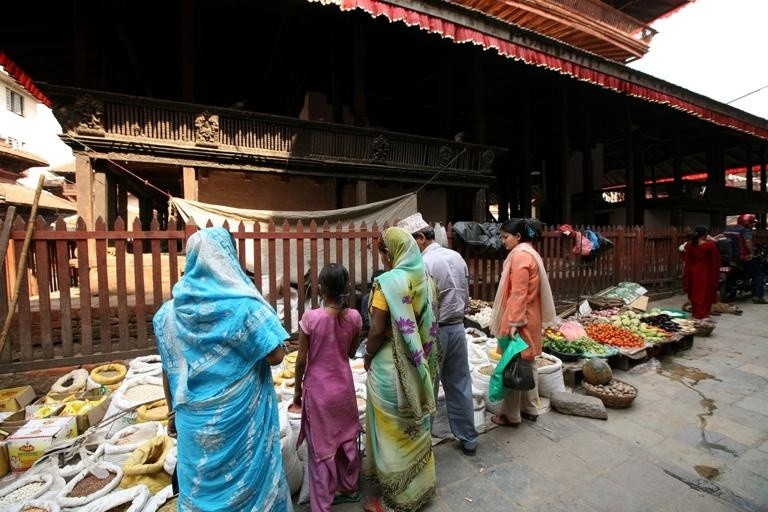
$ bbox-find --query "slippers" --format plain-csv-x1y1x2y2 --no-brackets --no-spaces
491,414,519,428
331,489,362,504
362,495,387,512
521,412,538,421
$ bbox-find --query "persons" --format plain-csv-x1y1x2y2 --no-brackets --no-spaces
361,269,387,338
398,213,481,457
360,225,440,512
679,212,767,321
489,219,558,427
294,262,364,510
153,226,295,511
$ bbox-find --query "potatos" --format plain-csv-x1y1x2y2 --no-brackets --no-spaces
585,379,637,397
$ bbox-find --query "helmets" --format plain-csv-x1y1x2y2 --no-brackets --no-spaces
737,213,757,229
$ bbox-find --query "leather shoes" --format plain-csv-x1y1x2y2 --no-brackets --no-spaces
752,298,767,304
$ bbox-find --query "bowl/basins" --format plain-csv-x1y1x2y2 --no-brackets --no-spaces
547,341,584,361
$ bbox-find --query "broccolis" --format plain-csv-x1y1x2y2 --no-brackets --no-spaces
611,310,641,331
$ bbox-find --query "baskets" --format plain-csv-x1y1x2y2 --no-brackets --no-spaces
580,378,638,410
694,323,713,337
543,328,565,342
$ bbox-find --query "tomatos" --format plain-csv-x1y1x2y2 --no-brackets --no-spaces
586,324,644,348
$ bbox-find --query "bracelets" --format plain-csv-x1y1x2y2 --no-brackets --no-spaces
167,410,175,418
363,345,373,358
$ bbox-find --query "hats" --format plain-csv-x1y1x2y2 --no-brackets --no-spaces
396,212,429,235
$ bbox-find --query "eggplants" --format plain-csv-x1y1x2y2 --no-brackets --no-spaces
640,314,683,332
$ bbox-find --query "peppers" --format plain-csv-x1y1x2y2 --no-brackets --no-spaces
550,339,586,354
